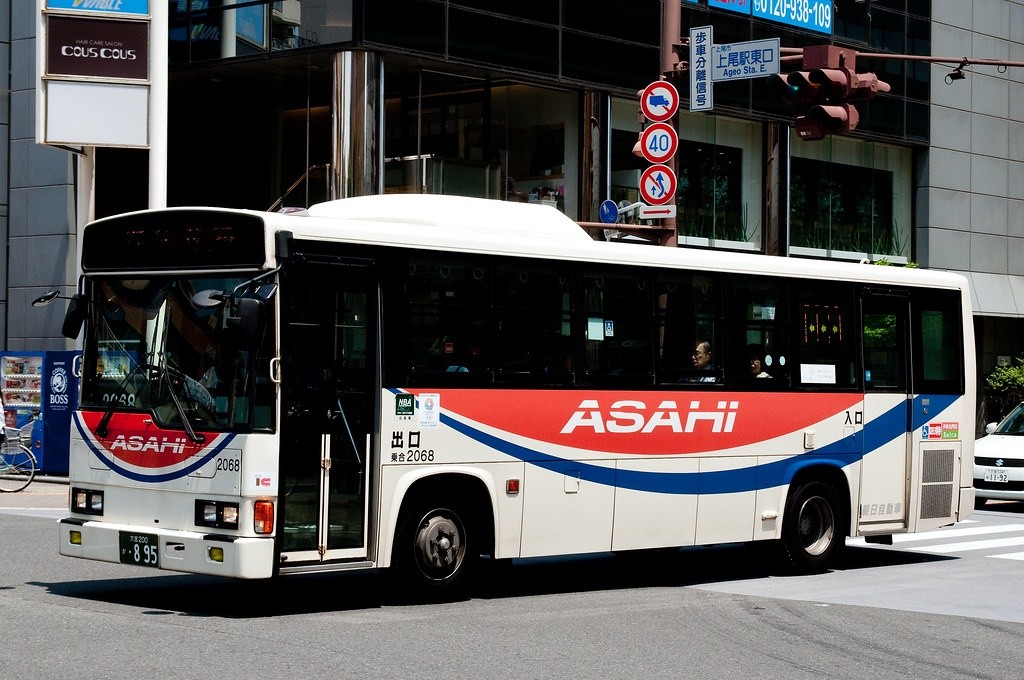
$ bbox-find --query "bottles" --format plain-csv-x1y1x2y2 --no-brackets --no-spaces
5,361,41,404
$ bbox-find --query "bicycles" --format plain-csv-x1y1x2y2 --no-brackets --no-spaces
0,427,38,494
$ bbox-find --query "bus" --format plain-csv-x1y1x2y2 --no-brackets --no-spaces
57,191,978,603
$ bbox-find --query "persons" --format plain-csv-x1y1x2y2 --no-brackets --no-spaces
741,348,781,390
0,398,6,449
678,340,720,384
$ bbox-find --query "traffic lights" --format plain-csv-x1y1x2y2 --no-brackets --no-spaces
809,103,859,135
770,67,858,104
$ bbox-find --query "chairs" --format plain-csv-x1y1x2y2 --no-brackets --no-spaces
492,367,786,390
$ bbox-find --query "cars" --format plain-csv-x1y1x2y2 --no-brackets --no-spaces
970,399,1024,510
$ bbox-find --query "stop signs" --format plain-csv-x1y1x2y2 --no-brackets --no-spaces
639,123,677,163
